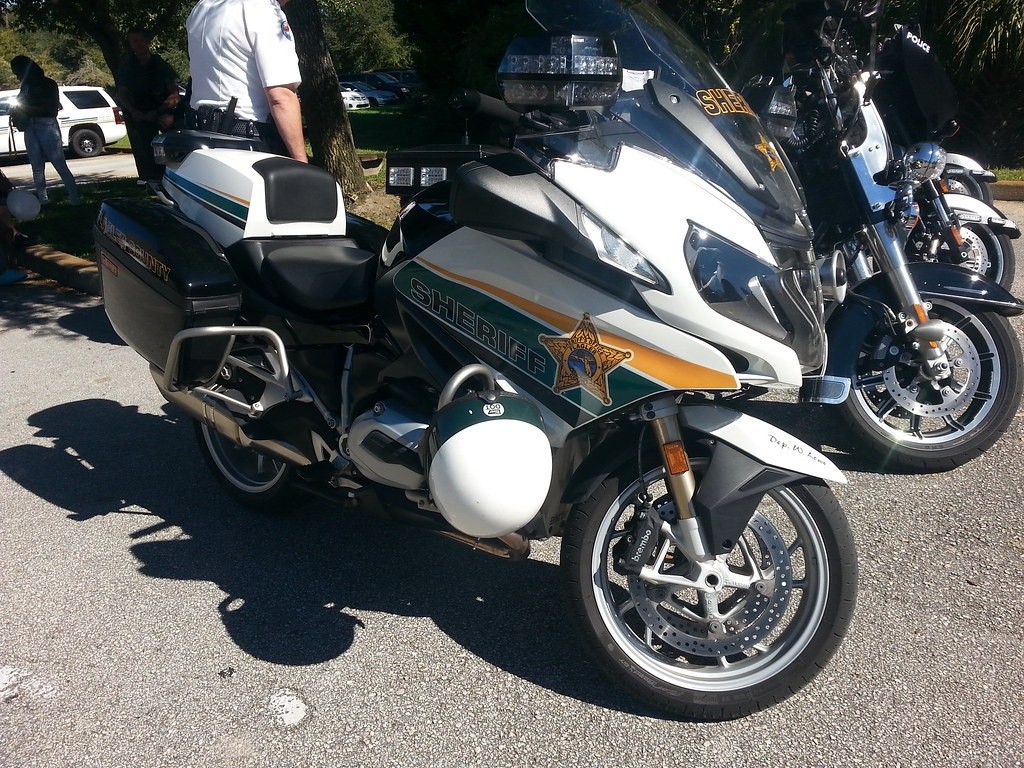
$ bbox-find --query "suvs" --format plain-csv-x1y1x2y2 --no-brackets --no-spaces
363,73,417,100
0,86,128,159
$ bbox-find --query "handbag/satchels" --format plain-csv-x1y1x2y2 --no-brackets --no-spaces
7,108,28,132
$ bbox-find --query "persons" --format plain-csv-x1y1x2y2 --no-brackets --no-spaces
10,55,83,214
184,0,308,163
0,172,28,286
115,29,181,179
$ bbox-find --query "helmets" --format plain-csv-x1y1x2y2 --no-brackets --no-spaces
421,388,553,539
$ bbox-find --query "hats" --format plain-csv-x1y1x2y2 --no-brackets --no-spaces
10,56,34,81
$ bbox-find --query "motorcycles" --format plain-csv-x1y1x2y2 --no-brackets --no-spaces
94,0,1024,722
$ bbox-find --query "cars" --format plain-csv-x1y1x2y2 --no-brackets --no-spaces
342,83,400,107
338,82,370,111
